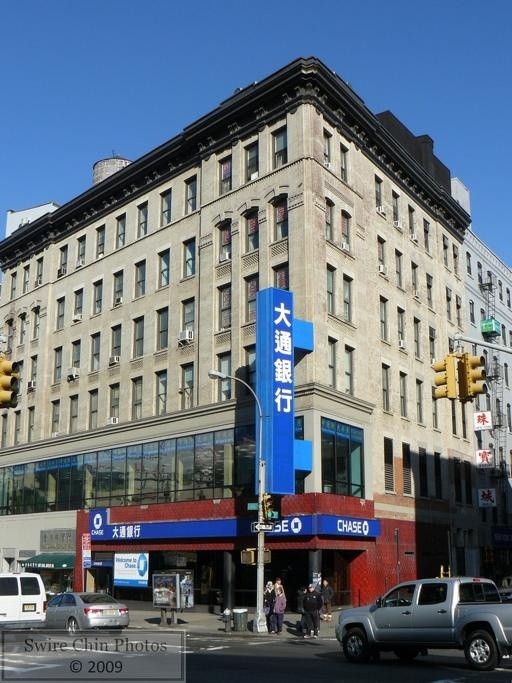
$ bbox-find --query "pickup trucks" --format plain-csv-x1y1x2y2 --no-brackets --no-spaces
335,578,512,671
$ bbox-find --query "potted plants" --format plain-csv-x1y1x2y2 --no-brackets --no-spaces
105,417,117,425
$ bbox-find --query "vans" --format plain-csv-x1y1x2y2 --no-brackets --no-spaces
0,571,46,628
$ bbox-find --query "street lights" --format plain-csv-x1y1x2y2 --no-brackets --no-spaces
208,369,269,634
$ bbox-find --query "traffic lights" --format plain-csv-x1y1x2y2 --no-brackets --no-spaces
0,357,20,408
430,353,487,402
261,492,273,520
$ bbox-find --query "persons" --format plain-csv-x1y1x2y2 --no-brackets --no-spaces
302,583,324,638
273,576,287,602
296,584,308,635
319,579,334,622
269,586,288,633
263,580,276,632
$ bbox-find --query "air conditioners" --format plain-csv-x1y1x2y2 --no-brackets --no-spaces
26,258,122,391
342,206,421,275
220,252,231,262
397,339,407,350
178,331,193,341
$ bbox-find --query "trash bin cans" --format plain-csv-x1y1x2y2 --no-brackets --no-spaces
233,609,248,631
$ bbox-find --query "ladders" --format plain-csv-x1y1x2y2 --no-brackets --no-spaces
440,565,451,578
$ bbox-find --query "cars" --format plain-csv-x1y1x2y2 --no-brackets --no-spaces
47,591,130,633
